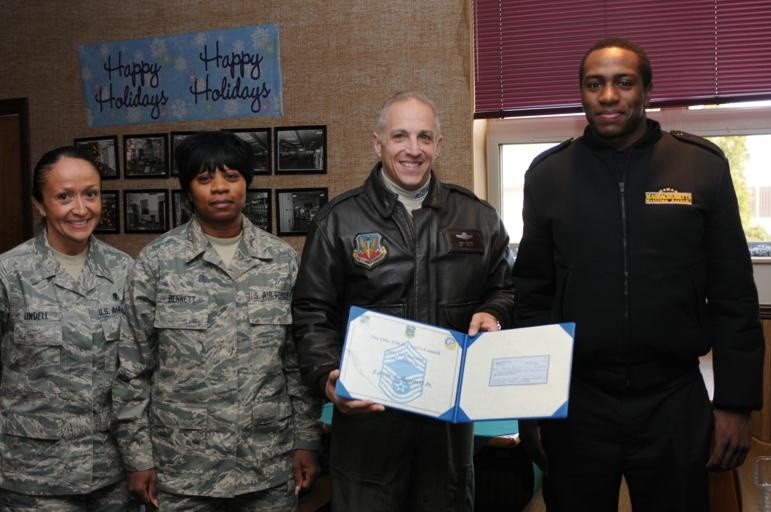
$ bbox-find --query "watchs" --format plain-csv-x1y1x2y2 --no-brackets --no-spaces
496,320,501,328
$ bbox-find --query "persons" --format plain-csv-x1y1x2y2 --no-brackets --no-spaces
0,146,144,512
109,129,323,511
290,91,517,511
509,36,764,510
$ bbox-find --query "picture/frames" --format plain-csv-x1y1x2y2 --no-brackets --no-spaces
71,123,329,238
